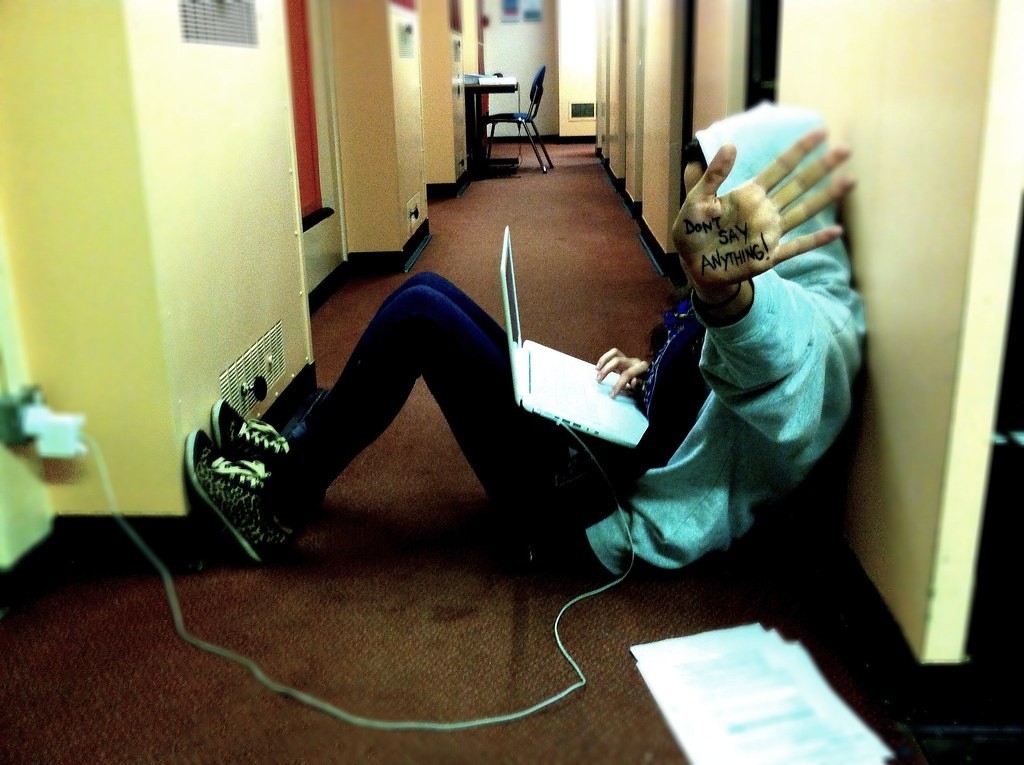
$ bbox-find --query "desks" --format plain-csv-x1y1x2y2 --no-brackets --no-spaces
465,84,521,180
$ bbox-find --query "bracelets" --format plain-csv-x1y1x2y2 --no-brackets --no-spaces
692,282,741,310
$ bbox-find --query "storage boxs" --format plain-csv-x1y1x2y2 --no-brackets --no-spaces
478,77,516,86
464,74,497,85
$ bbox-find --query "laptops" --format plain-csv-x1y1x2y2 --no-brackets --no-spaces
500,225,649,448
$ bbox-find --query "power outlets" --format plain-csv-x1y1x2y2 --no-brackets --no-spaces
0,384,48,448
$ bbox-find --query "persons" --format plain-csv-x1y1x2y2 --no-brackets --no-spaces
184,101,866,582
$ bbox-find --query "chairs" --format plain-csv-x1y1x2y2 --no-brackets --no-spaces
479,65,555,174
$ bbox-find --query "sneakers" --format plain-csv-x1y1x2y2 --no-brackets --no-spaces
213,399,319,524
187,428,290,562
